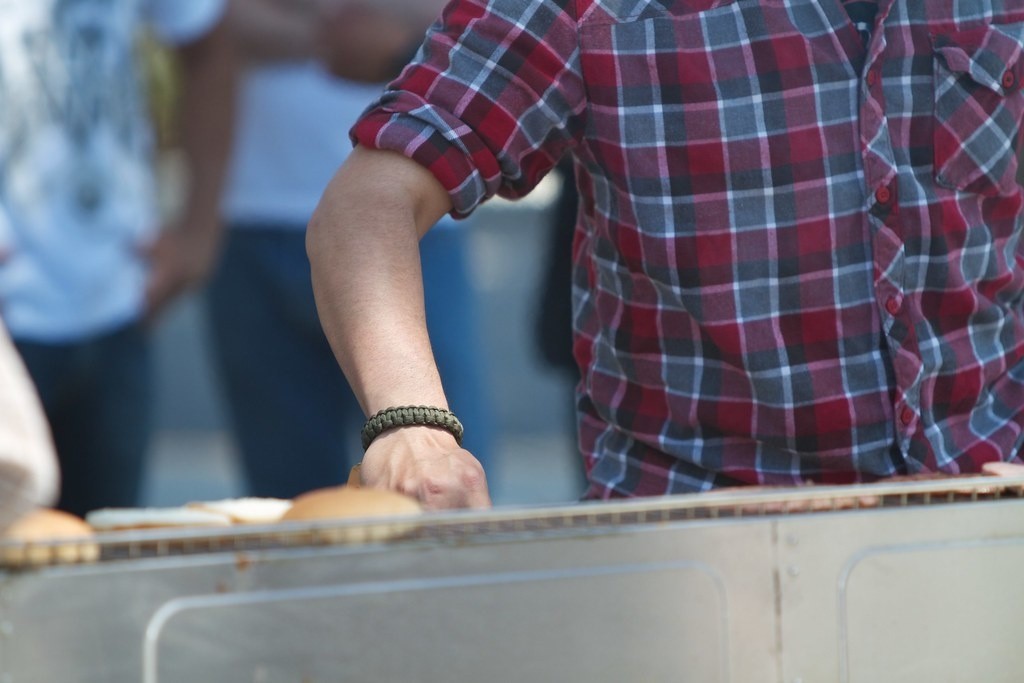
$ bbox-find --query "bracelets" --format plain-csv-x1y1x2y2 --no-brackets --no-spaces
360,404,463,453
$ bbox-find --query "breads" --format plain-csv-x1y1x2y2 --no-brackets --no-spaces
0,508,101,569
259,484,424,545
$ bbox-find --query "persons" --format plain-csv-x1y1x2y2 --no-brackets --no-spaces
0,0,496,523
305,0,1024,511
